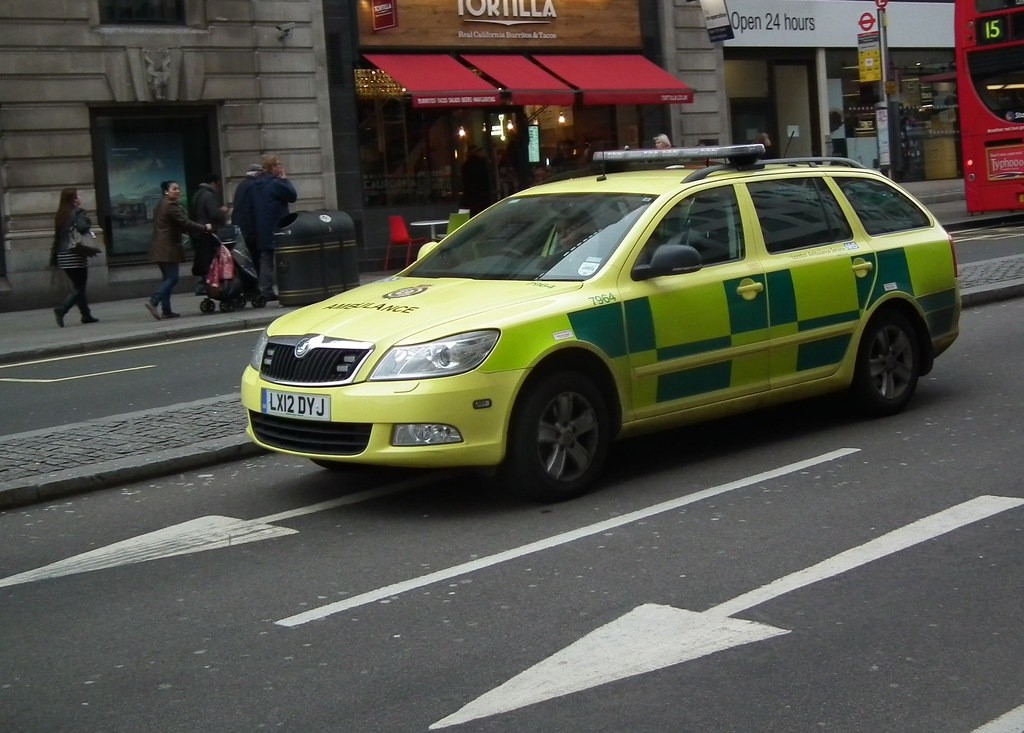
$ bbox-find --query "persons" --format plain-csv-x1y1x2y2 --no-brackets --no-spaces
192,154,297,301
146,180,213,320
53,188,99,329
464,126,585,217
651,133,779,161
539,207,598,267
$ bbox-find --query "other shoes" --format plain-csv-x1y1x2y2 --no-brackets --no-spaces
54,307,65,328
81,317,99,324
195,291,207,296
256,293,279,308
161,312,180,319
145,301,161,320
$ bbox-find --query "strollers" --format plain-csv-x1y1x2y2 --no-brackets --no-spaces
199,224,268,313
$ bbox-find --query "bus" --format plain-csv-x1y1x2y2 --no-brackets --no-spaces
954,0,1024,211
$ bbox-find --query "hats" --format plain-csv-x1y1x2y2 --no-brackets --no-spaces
249,164,263,171
653,134,671,147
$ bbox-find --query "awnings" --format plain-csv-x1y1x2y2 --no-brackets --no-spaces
361,53,694,109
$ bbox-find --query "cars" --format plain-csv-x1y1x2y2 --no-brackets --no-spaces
240,145,964,503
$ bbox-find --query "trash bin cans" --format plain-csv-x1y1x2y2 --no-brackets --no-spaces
272,208,363,308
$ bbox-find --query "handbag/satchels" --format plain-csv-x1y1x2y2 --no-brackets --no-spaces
68,209,103,258
